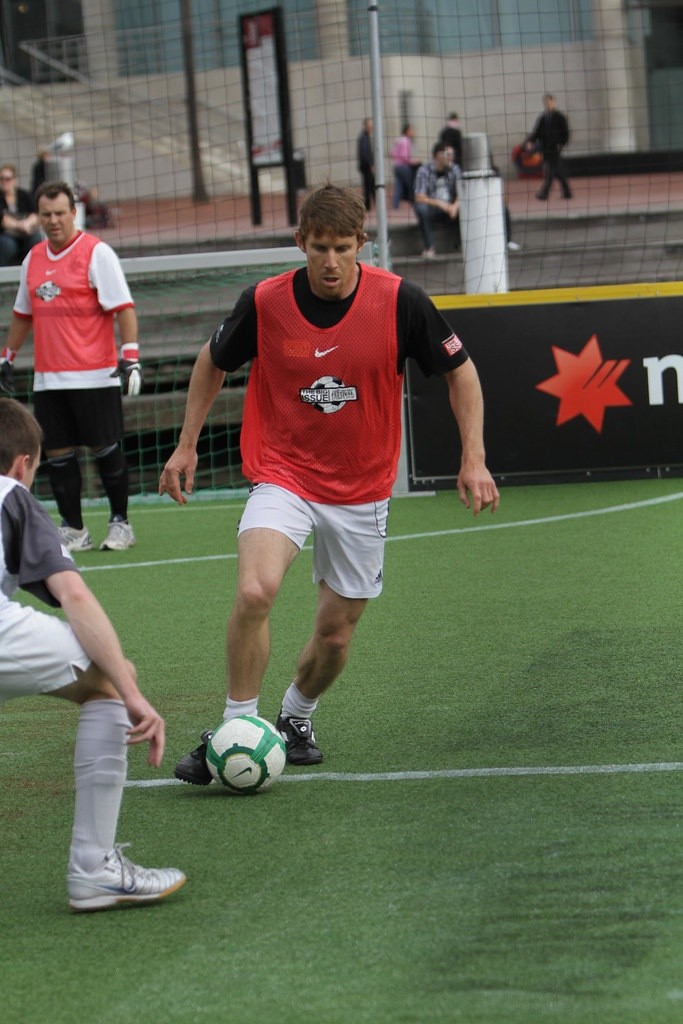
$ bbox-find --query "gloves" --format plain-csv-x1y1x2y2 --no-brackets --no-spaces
0,347,16,393
110,358,144,398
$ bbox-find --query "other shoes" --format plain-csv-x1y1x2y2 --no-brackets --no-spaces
421,246,436,259
508,241,521,250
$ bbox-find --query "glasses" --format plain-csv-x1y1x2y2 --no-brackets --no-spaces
1,176,16,182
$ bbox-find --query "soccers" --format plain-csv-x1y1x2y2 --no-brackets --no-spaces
205,713,288,795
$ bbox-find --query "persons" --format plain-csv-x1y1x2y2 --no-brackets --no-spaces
521,93,577,201
157,175,501,786
357,110,522,260
0,394,187,915
0,147,112,268
0,179,144,556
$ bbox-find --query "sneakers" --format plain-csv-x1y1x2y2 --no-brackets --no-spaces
99,521,136,552
173,730,214,786
65,844,186,910
56,526,95,552
277,706,326,767
